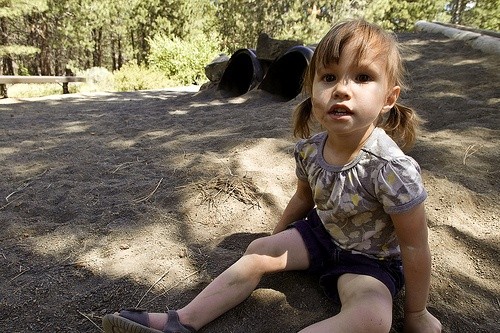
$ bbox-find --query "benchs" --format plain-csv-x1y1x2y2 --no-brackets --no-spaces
0,74,86,99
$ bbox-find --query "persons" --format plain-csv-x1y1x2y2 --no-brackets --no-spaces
101,22,443,333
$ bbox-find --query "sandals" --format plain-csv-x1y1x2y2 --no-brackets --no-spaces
98,305,196,333
